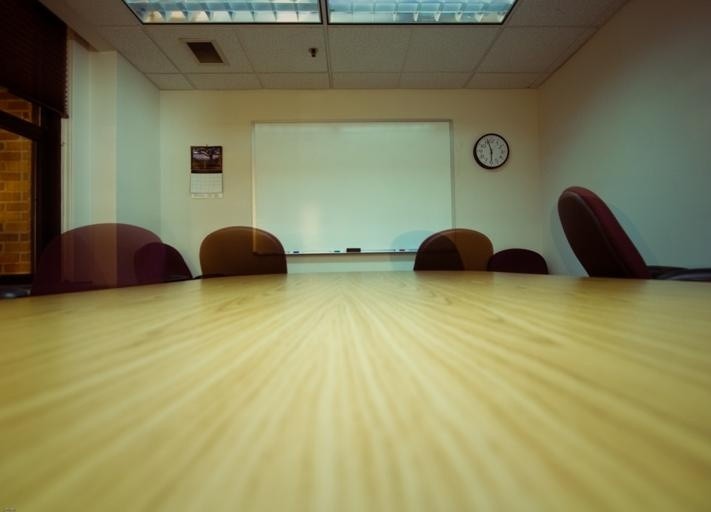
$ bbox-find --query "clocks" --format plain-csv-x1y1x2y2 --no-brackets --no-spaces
473,133,509,169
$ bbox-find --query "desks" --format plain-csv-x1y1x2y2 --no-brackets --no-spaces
0,271,711,512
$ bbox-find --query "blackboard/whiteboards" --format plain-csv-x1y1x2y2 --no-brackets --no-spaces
250,118,455,255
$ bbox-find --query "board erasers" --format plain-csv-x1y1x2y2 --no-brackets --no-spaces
347,248,361,252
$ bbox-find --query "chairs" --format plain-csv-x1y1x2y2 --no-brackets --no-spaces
558,187,711,282
413,229,548,274
31,223,287,296
